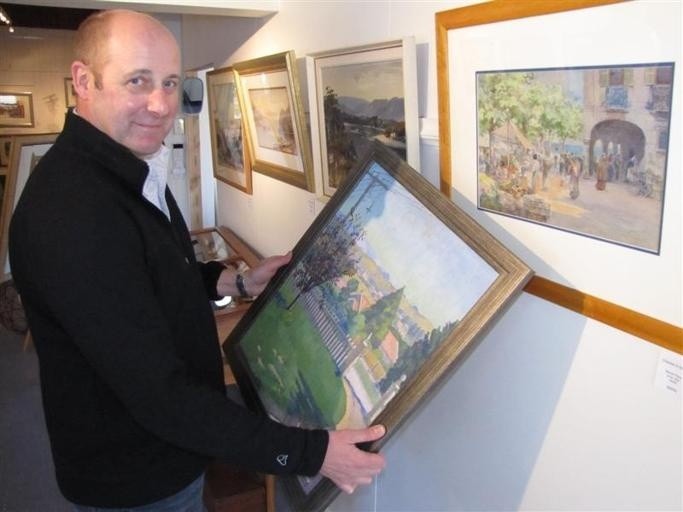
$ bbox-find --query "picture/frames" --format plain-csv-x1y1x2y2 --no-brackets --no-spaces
233,49,315,195
204,66,253,194
0,76,76,284
221,137,533,512
435,1,683,354
306,35,421,204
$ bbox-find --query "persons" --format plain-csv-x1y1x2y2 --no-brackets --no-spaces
6,8,387,511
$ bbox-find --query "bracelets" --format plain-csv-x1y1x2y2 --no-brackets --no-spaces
232,271,248,295
